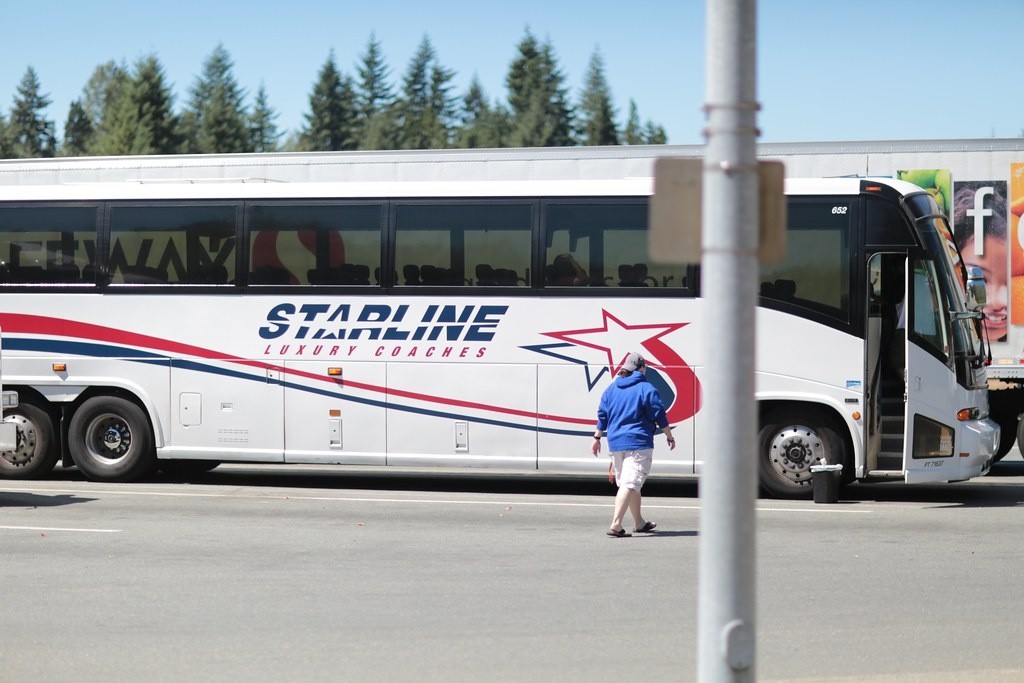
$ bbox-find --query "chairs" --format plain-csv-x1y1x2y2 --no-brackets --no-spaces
0,261,797,300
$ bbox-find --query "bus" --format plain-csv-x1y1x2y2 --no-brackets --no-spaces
0,179,1000,500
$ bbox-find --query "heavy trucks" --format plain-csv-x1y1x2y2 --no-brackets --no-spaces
0,137,1024,483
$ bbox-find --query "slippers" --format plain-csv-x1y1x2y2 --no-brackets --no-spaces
606,528,632,537
632,521,657,532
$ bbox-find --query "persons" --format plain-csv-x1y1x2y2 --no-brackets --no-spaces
591,351,675,537
954,186,1008,343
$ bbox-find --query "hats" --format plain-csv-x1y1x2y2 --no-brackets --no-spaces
621,352,645,371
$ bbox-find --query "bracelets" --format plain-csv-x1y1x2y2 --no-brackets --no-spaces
667,437,674,442
594,436,601,440
